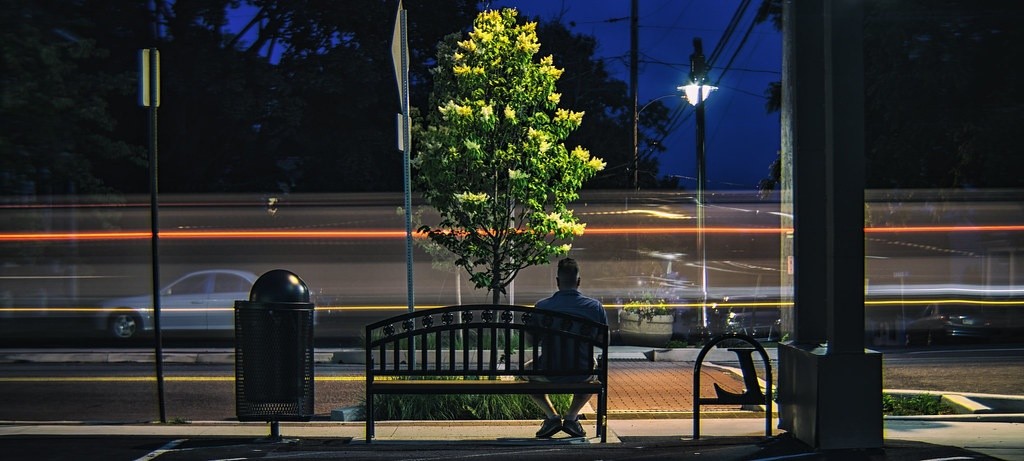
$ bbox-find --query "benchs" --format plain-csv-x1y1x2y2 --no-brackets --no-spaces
364,304,608,444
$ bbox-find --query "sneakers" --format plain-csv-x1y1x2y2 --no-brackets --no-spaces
561,415,588,436
535,413,562,438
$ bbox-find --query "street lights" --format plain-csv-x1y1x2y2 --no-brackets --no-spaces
626,80,722,300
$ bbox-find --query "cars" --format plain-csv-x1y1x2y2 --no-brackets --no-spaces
905,304,994,348
95,269,318,348
711,294,780,342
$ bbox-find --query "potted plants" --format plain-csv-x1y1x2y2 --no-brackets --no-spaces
619,297,674,348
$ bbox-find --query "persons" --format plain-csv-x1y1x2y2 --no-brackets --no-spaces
522,258,609,437
